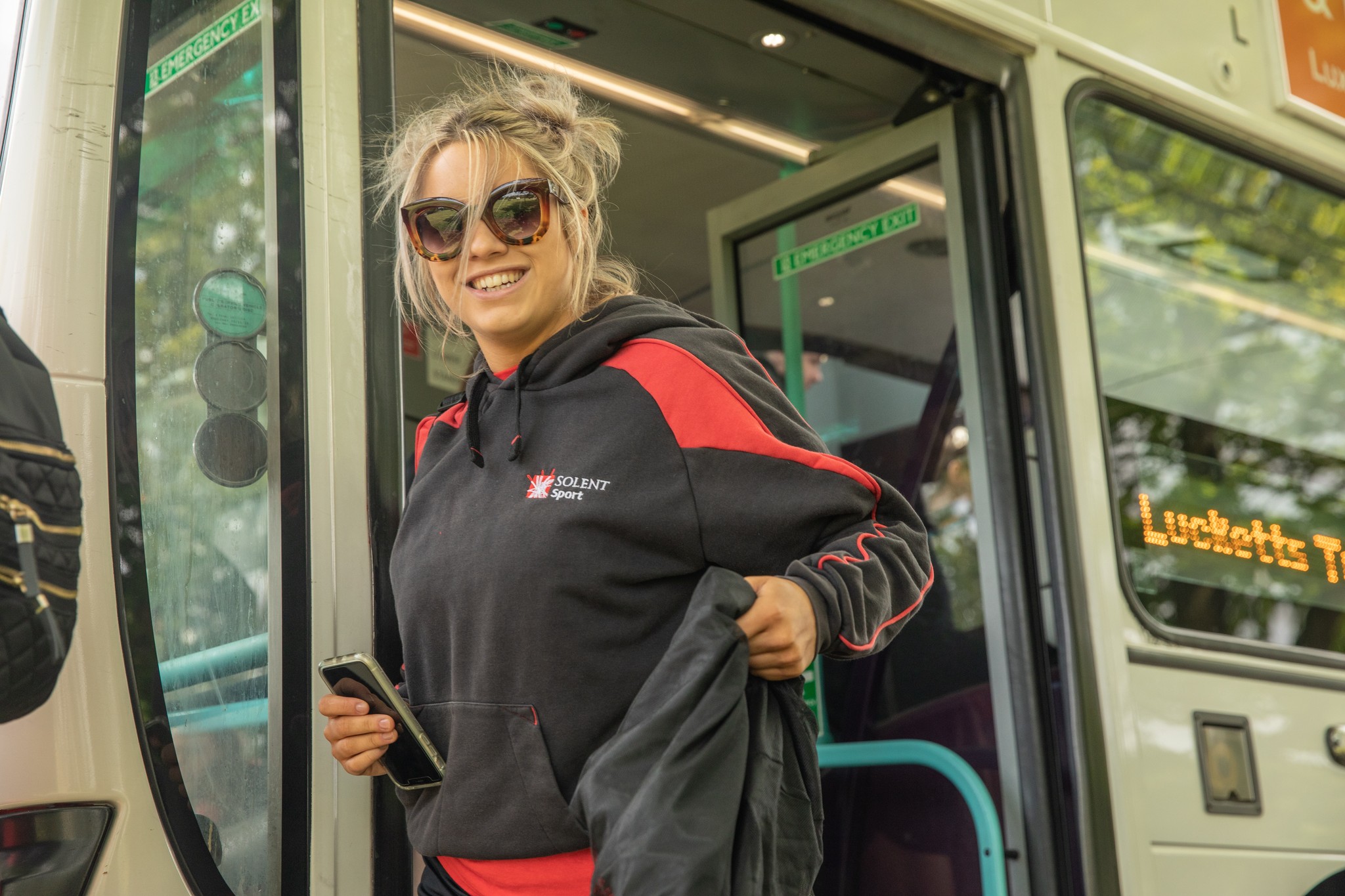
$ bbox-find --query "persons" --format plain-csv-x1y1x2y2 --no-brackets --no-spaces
319,55,935,896
764,348,826,397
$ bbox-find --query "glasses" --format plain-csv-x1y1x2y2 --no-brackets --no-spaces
398,178,572,263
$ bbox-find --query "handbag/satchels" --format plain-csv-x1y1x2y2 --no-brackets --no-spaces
0,311,84,724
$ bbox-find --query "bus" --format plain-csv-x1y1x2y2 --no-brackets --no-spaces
1,0,1344,896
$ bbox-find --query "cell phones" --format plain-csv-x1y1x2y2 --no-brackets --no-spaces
316,652,449,790
